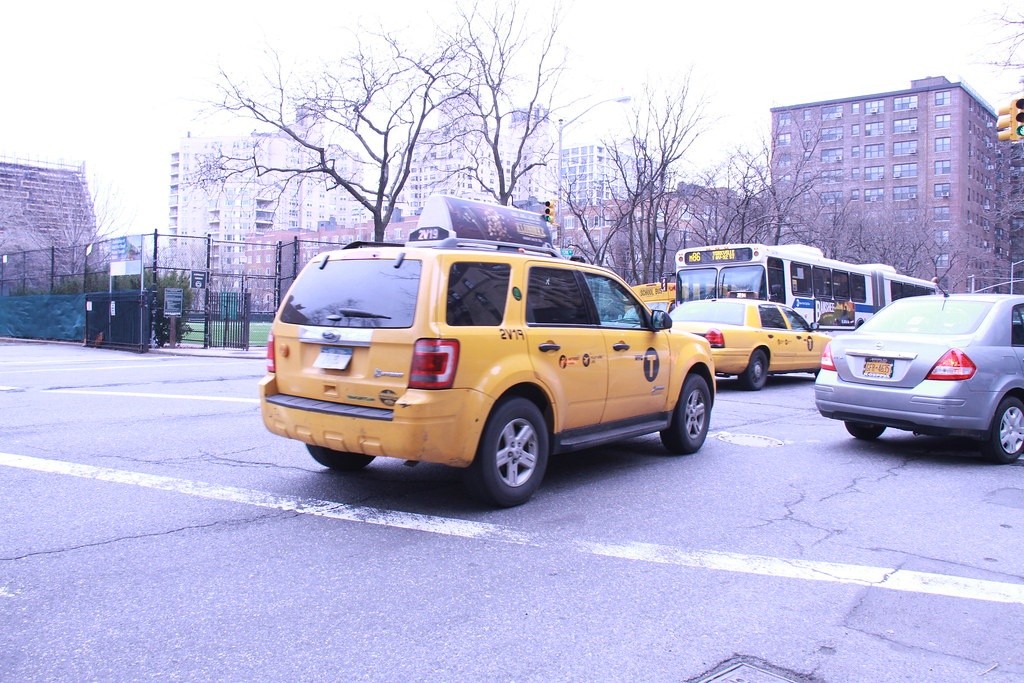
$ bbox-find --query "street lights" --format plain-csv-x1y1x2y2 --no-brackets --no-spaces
556,96,633,246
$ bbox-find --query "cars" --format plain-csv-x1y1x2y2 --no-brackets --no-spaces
623,280,737,330
657,291,837,388
813,292,1024,465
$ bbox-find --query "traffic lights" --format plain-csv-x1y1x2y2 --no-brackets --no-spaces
996,105,1013,140
1011,98,1024,142
543,199,557,223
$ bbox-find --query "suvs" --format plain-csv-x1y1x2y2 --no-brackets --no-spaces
259,194,722,509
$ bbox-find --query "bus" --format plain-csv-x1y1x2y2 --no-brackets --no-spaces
669,242,946,346
